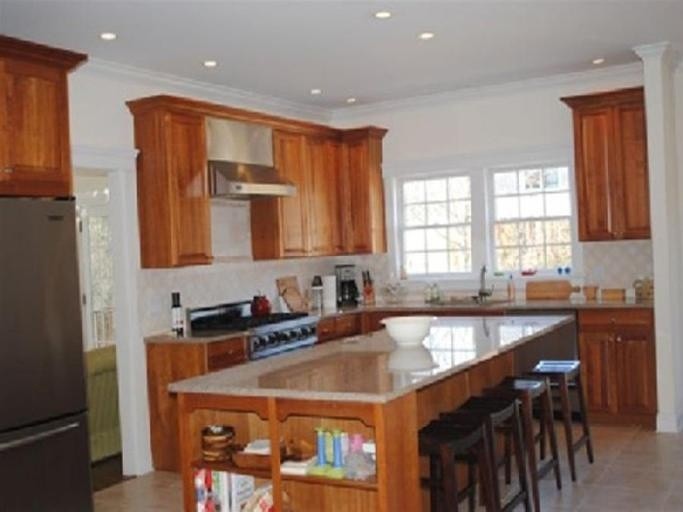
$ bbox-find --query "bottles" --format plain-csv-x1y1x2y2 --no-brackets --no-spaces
310,275,324,311
171,292,184,334
507,274,515,299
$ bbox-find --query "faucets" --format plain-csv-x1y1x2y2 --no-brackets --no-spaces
479,265,494,303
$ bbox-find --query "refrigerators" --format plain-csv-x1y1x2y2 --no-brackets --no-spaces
0,197,92,512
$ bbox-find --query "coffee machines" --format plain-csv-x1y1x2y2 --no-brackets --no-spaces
335,264,359,310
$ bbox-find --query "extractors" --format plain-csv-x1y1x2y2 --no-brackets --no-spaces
206,116,296,201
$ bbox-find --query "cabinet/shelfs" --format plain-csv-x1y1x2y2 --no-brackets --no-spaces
251,116,339,259
561,86,652,241
149,335,252,472
127,95,212,268
342,125,388,256
0,36,88,198
577,292,658,426
280,302,357,342
179,394,380,512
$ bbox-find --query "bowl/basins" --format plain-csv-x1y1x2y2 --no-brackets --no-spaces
379,316,438,348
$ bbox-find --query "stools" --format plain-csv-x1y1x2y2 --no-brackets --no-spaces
483,374,565,512
417,420,494,512
528,360,593,481
441,395,531,511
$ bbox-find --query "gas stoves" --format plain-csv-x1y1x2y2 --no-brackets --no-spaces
187,300,317,361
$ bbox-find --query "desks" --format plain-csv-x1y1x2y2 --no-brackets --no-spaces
167,315,579,512
